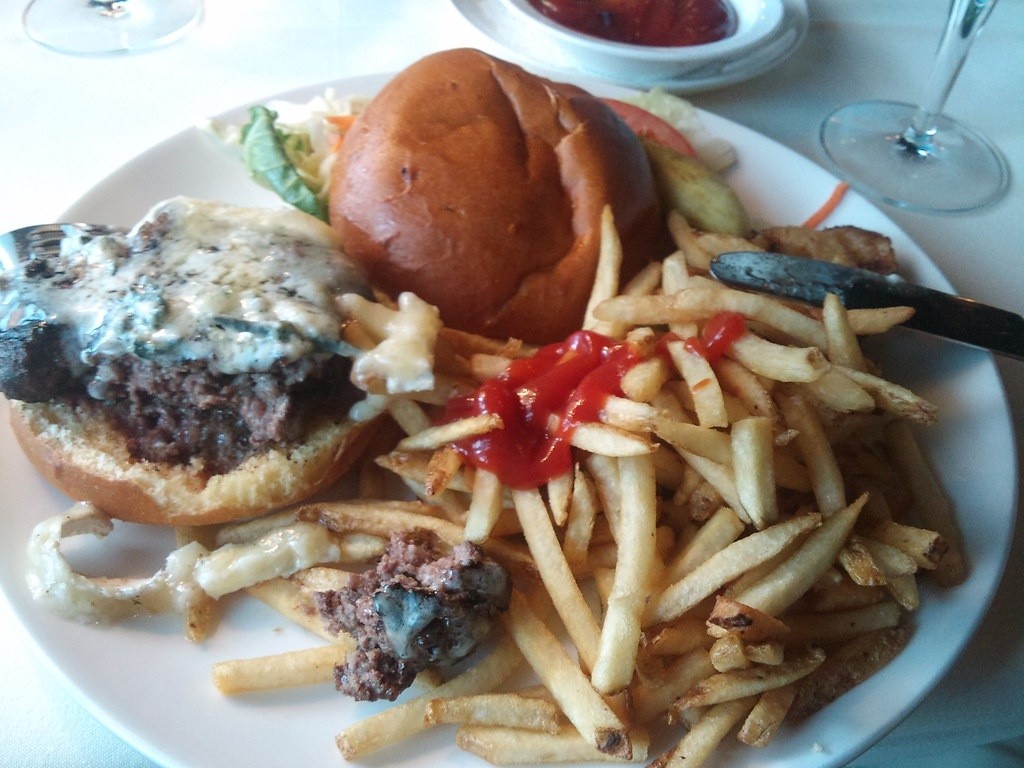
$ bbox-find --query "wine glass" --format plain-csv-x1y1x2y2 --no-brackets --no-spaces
21,0,205,56
822,0,1011,212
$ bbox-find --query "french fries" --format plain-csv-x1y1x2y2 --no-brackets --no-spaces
19,205,967,768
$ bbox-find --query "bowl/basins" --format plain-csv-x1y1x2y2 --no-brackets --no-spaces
503,0,784,80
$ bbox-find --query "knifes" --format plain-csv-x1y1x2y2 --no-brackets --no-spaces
712,251,1023,369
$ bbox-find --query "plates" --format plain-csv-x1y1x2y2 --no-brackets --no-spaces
0,71,1015,768
447,0,810,91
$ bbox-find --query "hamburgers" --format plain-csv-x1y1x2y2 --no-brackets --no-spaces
1,46,754,530
